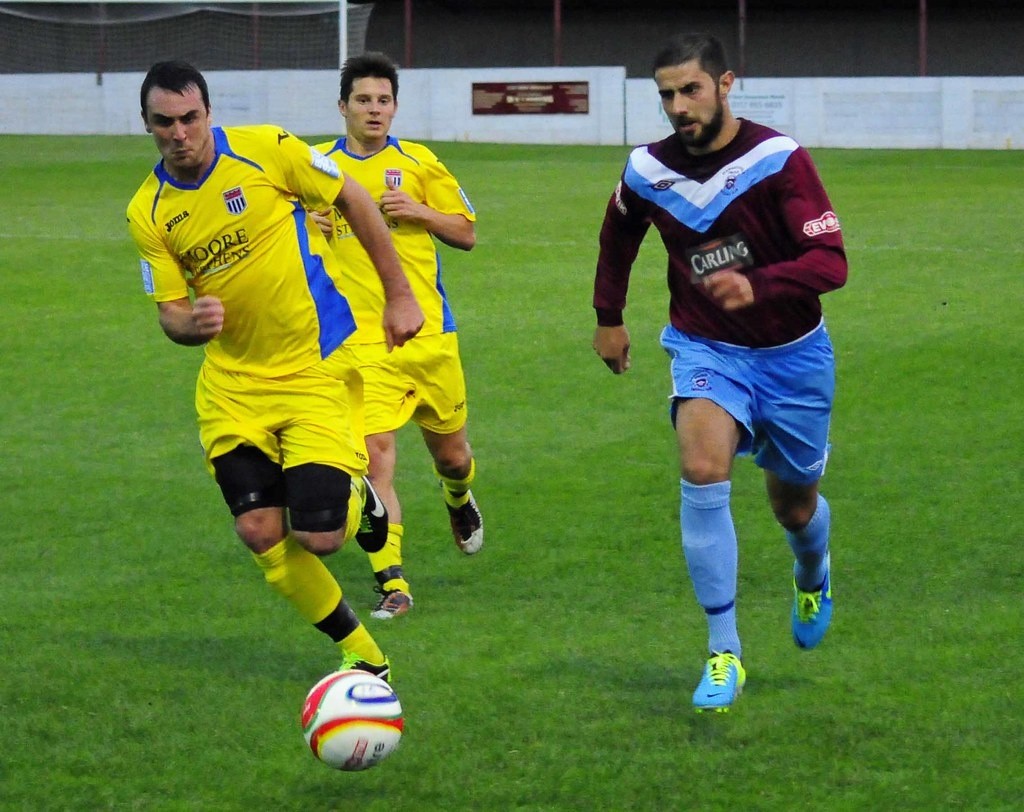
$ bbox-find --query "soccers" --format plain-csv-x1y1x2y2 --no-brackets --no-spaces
300,668,405,774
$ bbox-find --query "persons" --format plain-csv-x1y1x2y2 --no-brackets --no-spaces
308,52,485,617
124,59,426,686
590,35,847,716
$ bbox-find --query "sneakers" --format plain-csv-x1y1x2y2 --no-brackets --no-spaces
370,584,413,620
439,479,484,555
692,649,746,713
339,649,392,682
355,475,389,553
791,550,833,650
